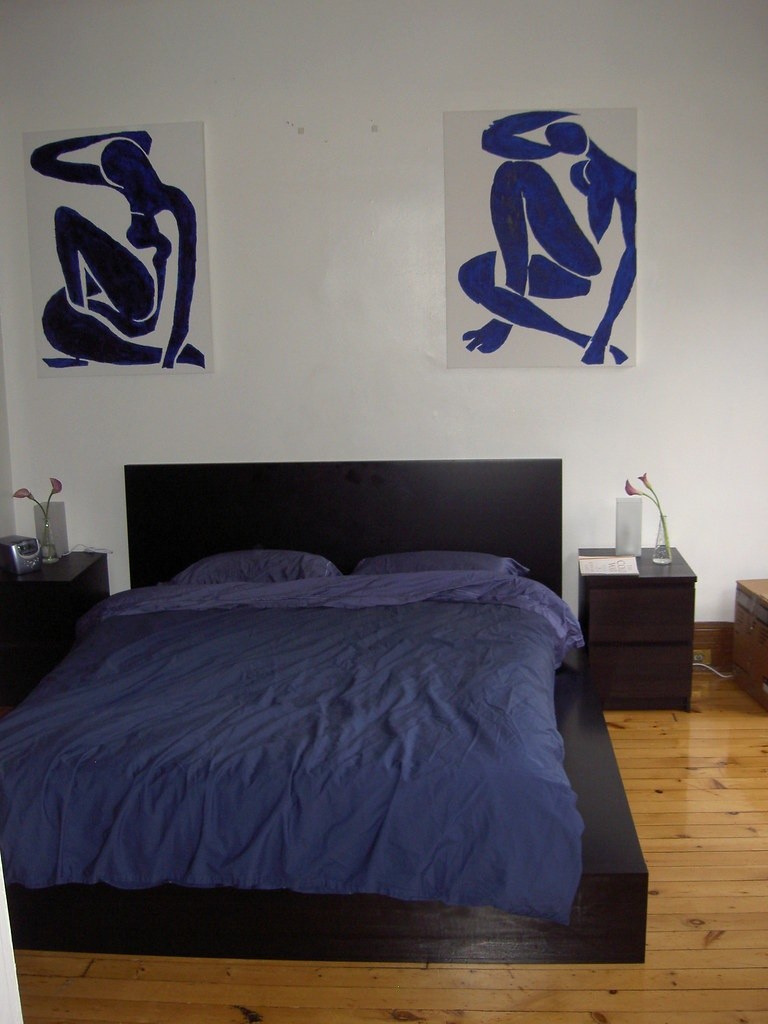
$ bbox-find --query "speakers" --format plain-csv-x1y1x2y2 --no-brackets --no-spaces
616,497,642,556
33,502,70,559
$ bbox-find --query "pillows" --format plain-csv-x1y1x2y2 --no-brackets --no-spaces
169,549,344,584
351,550,531,576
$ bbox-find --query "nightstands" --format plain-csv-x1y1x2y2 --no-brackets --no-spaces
578,548,698,712
0,552,110,708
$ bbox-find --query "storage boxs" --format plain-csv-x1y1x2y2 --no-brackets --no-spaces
731,579,768,710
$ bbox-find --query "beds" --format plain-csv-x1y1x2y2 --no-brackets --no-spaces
0,458,648,964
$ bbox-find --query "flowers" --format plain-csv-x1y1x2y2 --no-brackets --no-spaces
626,471,673,560
12,478,63,559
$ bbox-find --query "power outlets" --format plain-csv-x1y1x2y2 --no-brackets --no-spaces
693,649,712,666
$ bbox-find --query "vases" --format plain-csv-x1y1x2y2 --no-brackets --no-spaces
654,516,672,564
40,517,60,564
